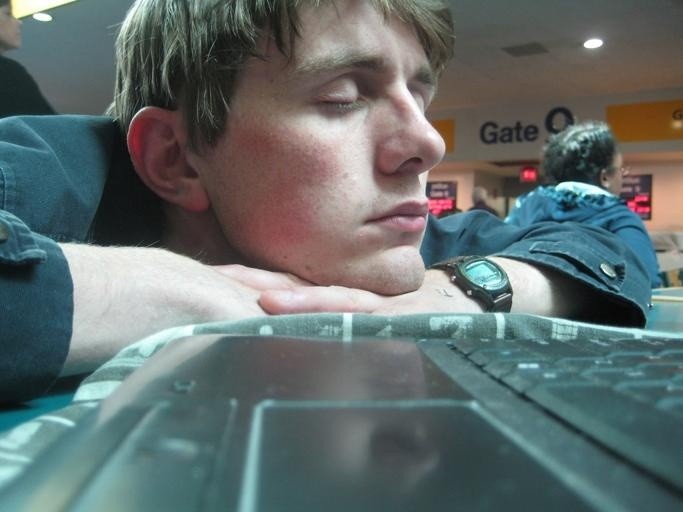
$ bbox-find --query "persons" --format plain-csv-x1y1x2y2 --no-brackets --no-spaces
0,1,662,418
466,187,498,218
541,117,633,203
0,1,59,125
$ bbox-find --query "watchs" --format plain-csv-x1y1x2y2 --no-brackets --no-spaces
428,251,513,313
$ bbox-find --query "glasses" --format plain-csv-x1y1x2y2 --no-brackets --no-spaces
612,167,631,178
